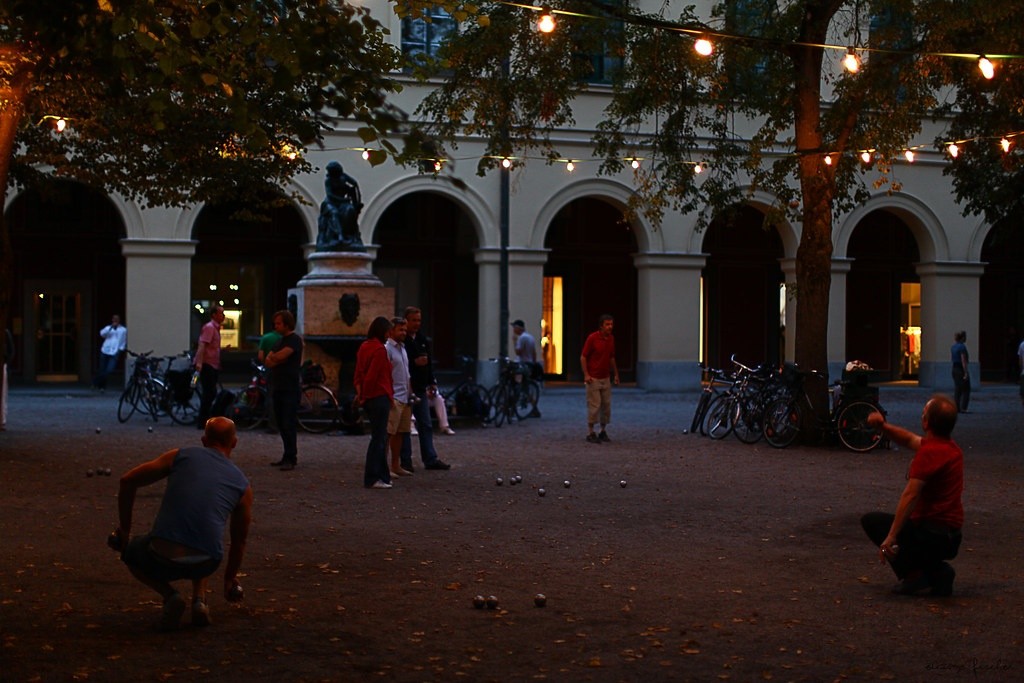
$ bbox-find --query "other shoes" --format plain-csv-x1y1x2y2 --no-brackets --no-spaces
264,428,278,435
933,561,956,597
190,599,212,628
424,459,451,470
368,478,394,489
198,422,205,430
269,455,295,472
527,412,541,418
400,464,414,473
100,387,105,393
598,430,611,442
396,468,414,477
586,432,602,444
156,593,186,634
410,428,419,436
961,409,973,414
896,575,923,596
443,428,456,435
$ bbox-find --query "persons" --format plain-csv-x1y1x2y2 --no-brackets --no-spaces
108,416,253,624
353,316,395,489
256,310,304,470
1018,341,1024,405
96,314,127,394
317,162,364,249
579,315,619,442
384,317,414,479
510,320,541,417
860,396,965,596
950,329,972,414
411,377,455,436
192,304,226,430
400,306,450,472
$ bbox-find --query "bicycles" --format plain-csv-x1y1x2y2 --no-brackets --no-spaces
437,356,544,429
690,354,890,453
238,358,341,433
118,349,224,426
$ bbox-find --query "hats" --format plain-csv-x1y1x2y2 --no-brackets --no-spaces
509,319,524,327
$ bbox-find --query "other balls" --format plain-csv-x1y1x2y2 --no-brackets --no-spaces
96,467,104,475
472,595,485,609
537,488,546,497
509,475,522,486
147,426,153,433
619,480,627,488
534,593,546,606
227,583,244,599
487,595,499,609
563,480,571,488
105,468,112,476
495,477,504,486
86,469,94,477
95,427,102,434
683,428,688,434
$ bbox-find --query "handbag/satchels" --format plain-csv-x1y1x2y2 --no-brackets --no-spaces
299,358,326,386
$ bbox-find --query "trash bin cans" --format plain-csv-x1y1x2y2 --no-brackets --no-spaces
842,368,879,443
